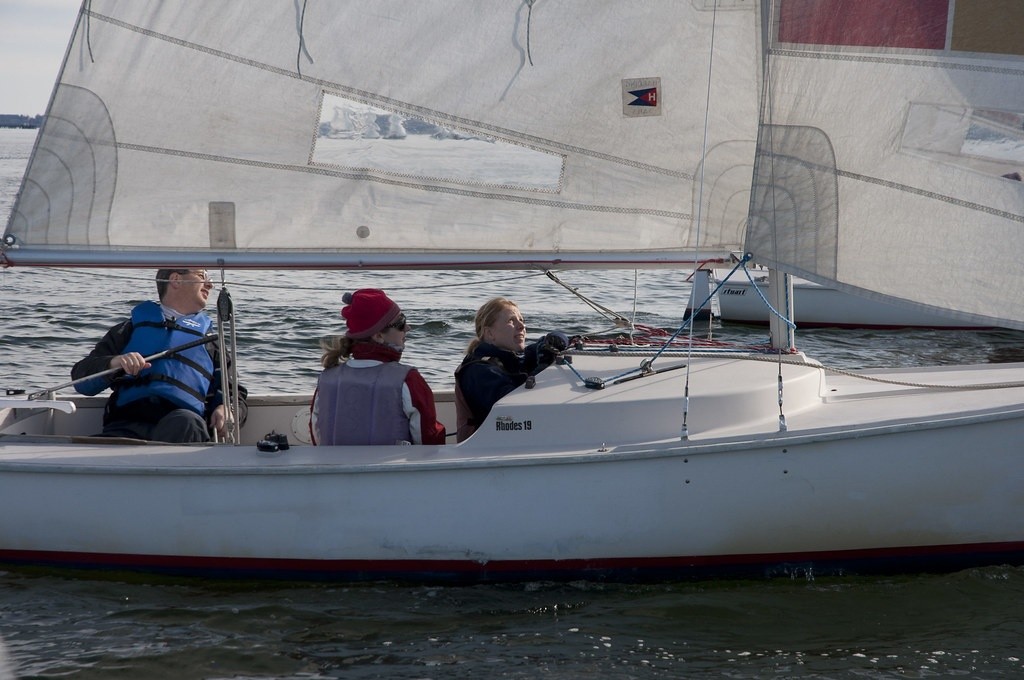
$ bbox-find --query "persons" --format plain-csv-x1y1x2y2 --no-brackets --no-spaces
312,288,447,445
455,298,569,443
72,268,246,445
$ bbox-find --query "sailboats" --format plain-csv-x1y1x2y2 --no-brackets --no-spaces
1,2,1021,574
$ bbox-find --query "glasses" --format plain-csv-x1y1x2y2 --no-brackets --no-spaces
386,313,406,331
184,271,213,285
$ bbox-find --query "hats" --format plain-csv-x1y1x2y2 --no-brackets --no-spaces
341,288,400,339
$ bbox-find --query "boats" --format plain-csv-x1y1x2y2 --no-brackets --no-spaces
711,267,1022,332
